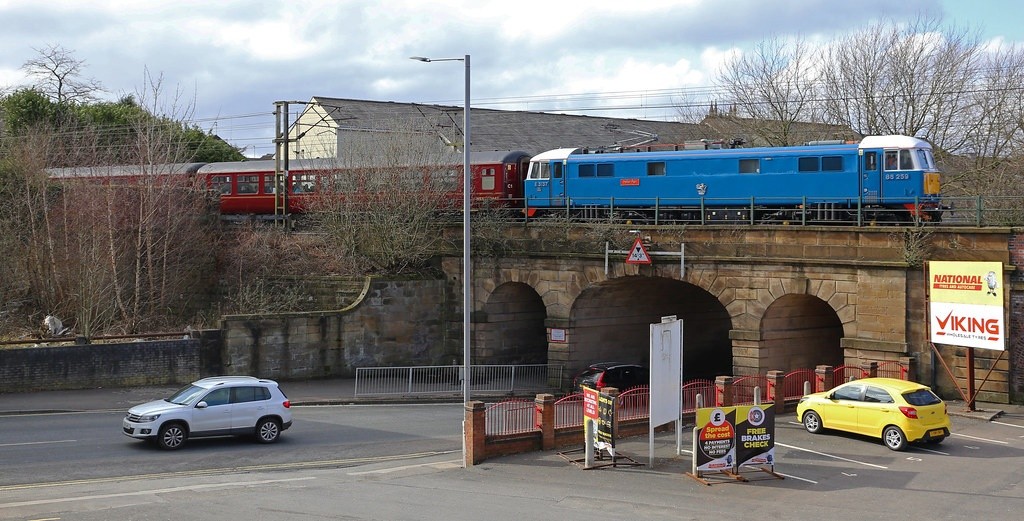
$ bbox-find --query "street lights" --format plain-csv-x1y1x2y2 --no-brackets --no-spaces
408,54,470,468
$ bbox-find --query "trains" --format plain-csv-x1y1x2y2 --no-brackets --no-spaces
35,121,941,225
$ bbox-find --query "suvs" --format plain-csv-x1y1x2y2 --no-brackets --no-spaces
122,375,293,451
574,362,650,391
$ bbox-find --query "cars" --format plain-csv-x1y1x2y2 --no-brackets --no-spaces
796,377,951,451
